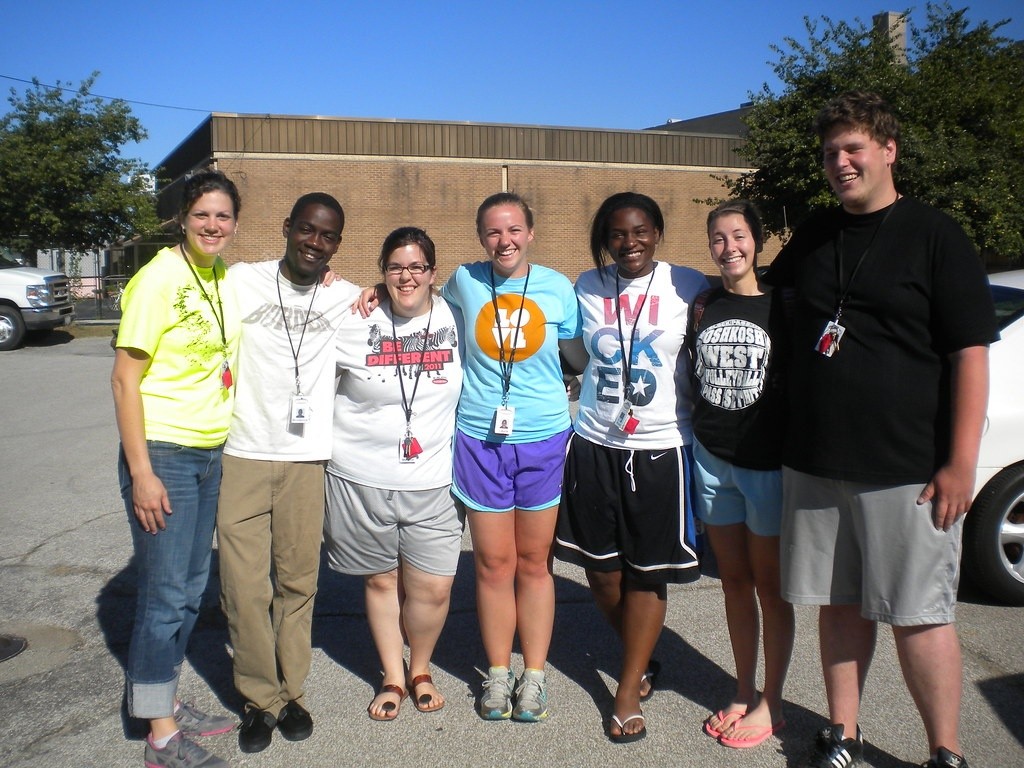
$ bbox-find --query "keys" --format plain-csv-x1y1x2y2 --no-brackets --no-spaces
819,332,839,355
405,438,412,455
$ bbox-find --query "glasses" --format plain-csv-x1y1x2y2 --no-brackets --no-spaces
385,262,431,274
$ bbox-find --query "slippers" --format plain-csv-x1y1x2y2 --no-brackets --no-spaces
639,660,661,702
409,674,445,712
705,704,753,739
610,709,647,743
369,685,411,721
720,717,786,748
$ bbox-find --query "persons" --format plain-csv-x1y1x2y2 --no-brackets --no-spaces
352,190,590,723
111,192,368,752
759,90,1001,768
111,170,343,768
686,199,795,749
501,420,508,428
562,190,711,743
333,224,586,721
296,409,304,417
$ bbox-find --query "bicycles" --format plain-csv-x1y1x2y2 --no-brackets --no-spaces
103,282,125,311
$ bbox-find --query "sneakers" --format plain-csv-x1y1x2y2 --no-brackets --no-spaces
237,708,277,752
513,669,548,722
480,665,518,720
277,700,313,742
174,700,235,737
145,730,232,768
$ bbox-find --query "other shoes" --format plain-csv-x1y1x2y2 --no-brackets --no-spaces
808,721,864,768
926,745,969,768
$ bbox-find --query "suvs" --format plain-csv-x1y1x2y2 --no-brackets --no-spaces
953,268,1024,607
0,240,75,351
